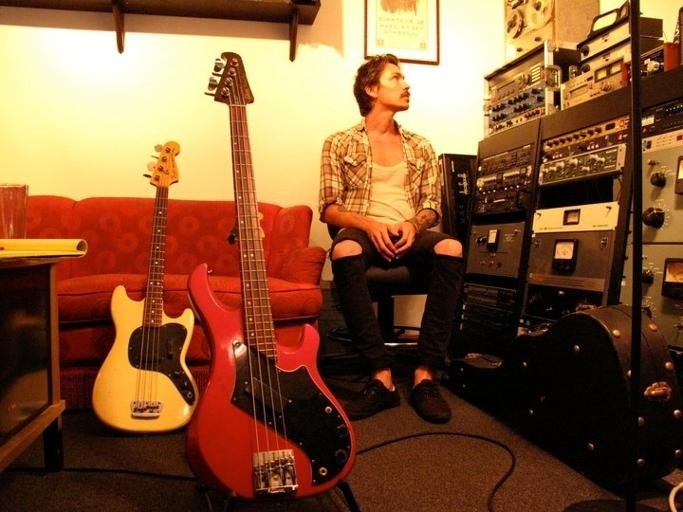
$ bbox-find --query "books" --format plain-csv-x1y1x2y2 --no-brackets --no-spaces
1,237,90,258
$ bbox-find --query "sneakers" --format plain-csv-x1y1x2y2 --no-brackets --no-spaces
344,378,398,421
409,378,453,423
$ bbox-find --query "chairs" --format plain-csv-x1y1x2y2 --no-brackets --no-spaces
326,223,431,388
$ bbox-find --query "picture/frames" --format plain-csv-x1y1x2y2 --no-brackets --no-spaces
363,1,440,66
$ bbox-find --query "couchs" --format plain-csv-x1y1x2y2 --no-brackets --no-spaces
1,184,328,412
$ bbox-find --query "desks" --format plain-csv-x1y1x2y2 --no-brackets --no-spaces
1,251,76,477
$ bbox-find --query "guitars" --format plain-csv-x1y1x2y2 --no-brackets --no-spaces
184,52,356,500
91,140,200,433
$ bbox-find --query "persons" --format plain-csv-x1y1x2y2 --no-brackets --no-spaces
316,51,467,425
